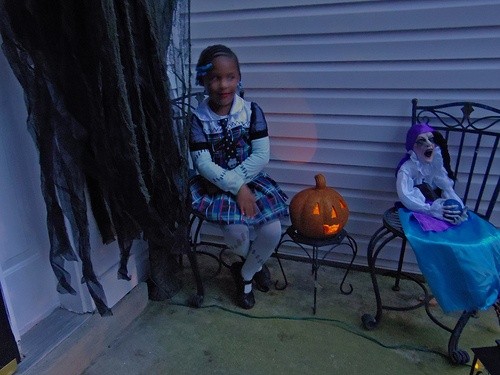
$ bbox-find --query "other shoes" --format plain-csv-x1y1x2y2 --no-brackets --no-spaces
242,243,271,292
232,262,255,310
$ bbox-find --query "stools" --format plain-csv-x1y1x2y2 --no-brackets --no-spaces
274,225,359,318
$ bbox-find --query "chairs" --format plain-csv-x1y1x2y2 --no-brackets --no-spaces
361,97,500,366
169,89,273,309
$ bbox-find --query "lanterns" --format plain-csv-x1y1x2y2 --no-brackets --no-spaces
289,174,349,238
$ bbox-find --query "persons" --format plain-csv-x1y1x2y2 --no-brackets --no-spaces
396,123,471,225
186,44,284,309
